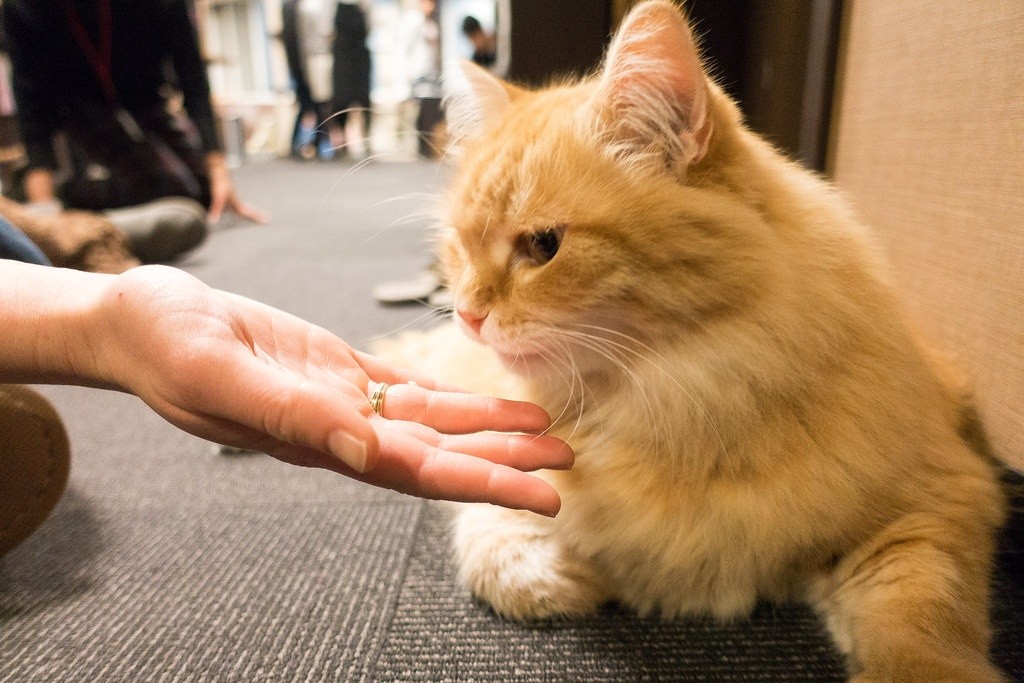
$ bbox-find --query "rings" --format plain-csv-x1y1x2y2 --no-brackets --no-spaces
370,382,389,417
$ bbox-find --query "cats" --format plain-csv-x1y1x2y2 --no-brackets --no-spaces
212,0,1005,683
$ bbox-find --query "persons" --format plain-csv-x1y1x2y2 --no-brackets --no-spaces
0,194,576,560
463,17,495,67
0,0,271,224
280,0,373,155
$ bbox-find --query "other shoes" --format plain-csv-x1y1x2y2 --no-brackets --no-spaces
374,264,443,302
96,198,208,264
428,287,455,312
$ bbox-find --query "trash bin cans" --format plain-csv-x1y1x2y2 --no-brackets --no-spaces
416,96,445,157
220,117,246,171
